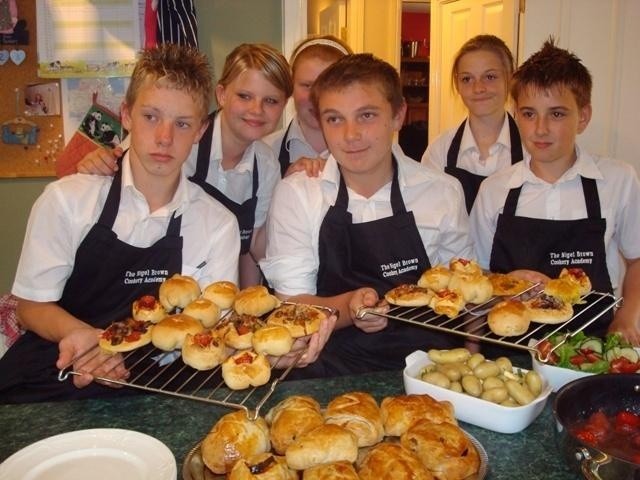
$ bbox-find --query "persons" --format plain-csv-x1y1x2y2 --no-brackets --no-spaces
1,41,242,401
462,40,638,371
417,32,530,220
247,33,357,297
59,39,328,293
258,53,487,383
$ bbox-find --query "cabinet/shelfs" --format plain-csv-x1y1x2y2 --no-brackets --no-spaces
400,56,429,125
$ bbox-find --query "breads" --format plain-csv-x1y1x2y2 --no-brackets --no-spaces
485,273,526,297
286,424,359,471
132,296,166,323
222,350,271,390
214,314,268,350
267,304,327,338
201,281,240,311
252,325,292,356
487,299,530,337
384,284,432,308
544,279,580,304
159,274,201,313
183,299,221,329
526,294,574,324
449,271,493,305
201,410,270,474
380,394,454,436
228,453,299,480
324,392,384,448
401,418,481,480
449,257,484,276
99,319,154,352
182,331,226,372
152,314,206,352
265,396,324,455
234,285,276,317
559,267,592,296
418,266,452,293
303,460,358,480
428,288,465,318
359,442,434,480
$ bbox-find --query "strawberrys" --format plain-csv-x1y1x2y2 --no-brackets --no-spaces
571,410,640,466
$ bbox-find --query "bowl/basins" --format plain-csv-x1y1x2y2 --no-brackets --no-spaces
552,373,640,480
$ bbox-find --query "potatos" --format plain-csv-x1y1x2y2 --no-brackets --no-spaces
418,348,541,408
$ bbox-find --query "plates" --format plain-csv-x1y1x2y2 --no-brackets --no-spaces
0,428,179,480
182,423,492,479
403,350,550,435
524,338,640,395
